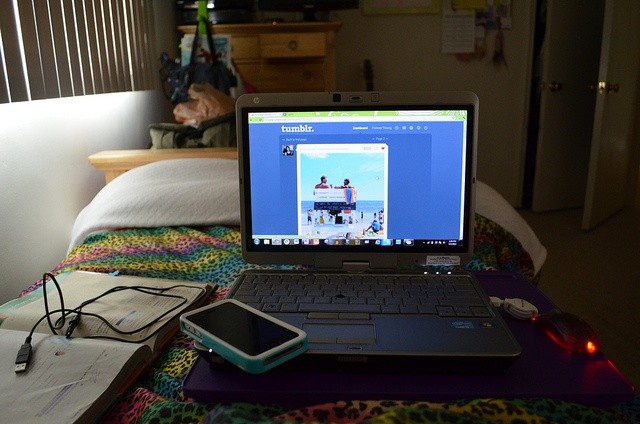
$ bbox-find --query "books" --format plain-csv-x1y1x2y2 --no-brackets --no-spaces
0,267,220,424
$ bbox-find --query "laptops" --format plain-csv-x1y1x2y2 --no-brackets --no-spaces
192,92,521,357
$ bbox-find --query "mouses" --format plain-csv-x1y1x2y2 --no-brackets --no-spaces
539,313,601,359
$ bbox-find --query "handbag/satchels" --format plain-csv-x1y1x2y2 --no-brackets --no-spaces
159,16,237,103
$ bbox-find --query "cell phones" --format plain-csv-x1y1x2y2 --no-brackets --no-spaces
178,298,309,375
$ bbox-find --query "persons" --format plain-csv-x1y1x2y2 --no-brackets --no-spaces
284,145,293,155
366,209,384,233
334,179,355,188
315,176,331,188
306,209,358,224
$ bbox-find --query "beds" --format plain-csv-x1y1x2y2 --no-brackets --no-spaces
11,146,640,424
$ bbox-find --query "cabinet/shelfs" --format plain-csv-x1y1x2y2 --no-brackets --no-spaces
177,21,338,93
